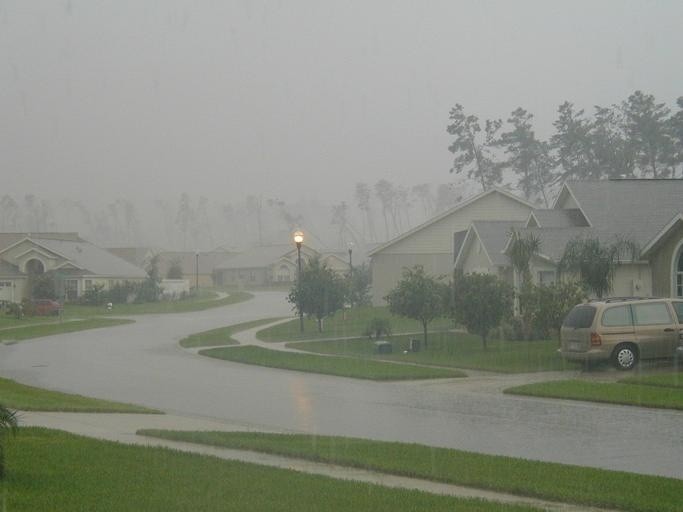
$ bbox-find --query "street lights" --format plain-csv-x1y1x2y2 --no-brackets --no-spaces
195,249,199,289
347,241,353,308
293,230,304,334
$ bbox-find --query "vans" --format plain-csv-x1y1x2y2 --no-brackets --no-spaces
555,295,681,370
23,300,62,316
